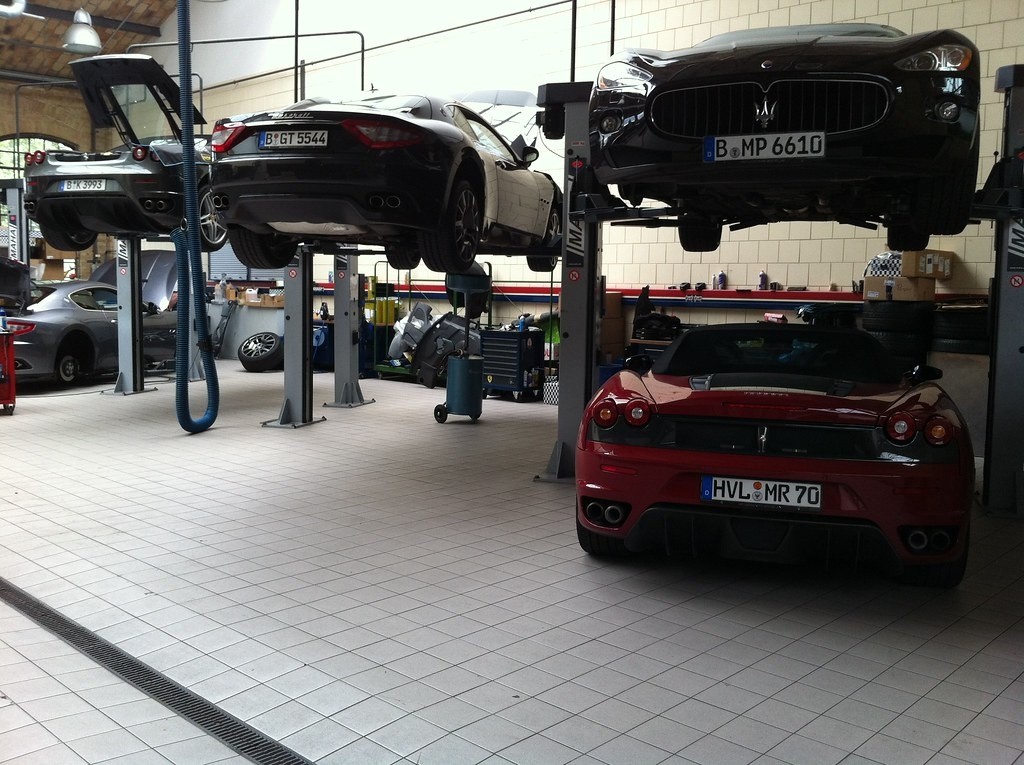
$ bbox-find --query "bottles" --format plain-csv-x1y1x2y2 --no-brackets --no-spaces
712,273,716,289
320,302,328,320
758,270,766,290
329,271,334,284
0,307,8,330
719,270,725,290
523,368,536,388
519,314,525,332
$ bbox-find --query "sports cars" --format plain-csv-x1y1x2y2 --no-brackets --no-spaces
574,319,975,589
212,91,562,274
22,52,229,253
0,250,178,393
586,22,983,254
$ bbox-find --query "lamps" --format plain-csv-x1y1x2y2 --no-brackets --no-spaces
60,0,103,53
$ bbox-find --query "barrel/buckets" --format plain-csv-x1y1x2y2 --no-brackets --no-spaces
246,289,257,302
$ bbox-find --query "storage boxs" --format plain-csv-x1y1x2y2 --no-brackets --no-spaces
544,367,559,405
630,338,672,360
863,250,953,302
29,232,114,278
204,286,285,308
600,290,624,363
